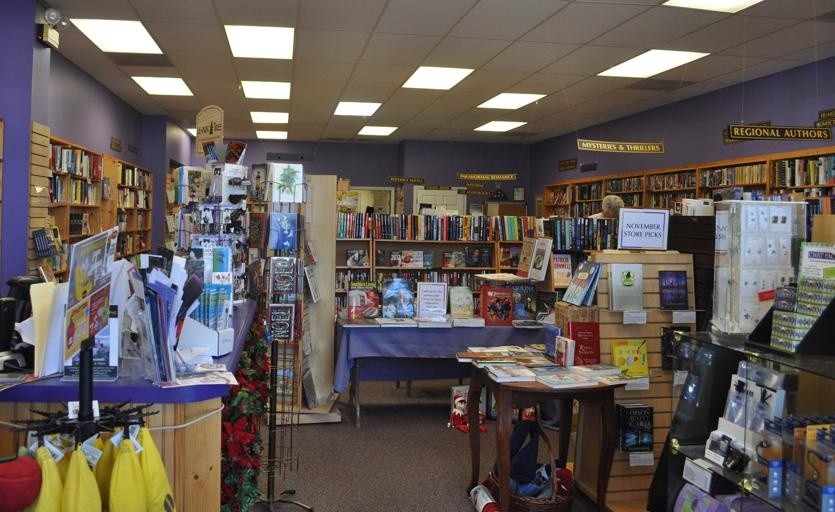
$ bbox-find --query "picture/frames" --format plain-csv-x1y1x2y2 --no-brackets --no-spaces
347,186,395,215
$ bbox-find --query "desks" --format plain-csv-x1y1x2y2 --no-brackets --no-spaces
334,318,548,429
454,346,627,512
1,299,258,512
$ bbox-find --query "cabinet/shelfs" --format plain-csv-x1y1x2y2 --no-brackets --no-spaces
540,145,835,253
336,240,556,316
646,331,835,512
48,135,154,285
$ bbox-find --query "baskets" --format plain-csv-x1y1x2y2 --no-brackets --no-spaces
489,424,575,512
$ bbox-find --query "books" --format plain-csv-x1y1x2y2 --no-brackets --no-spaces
606,263,691,371
456,322,650,391
550,154,835,218
46,142,202,261
336,213,620,327
